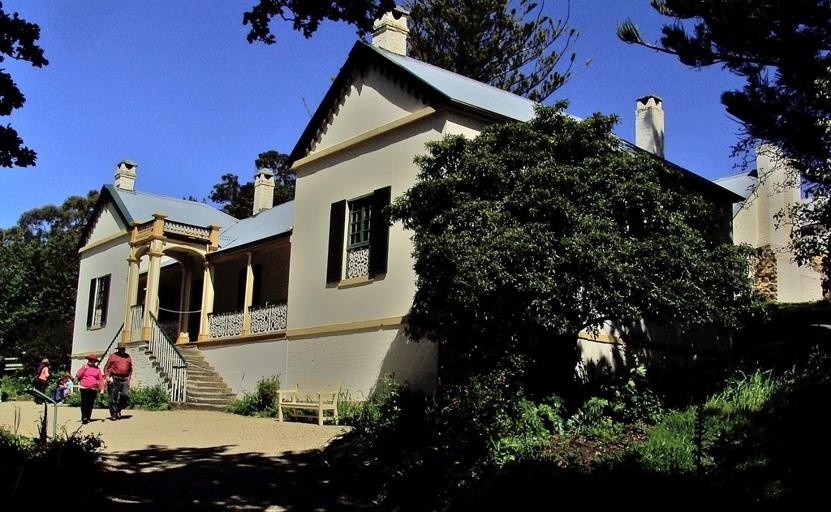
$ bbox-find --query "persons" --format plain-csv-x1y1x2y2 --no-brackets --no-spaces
74,353,105,424
34,358,51,405
61,375,74,403
52,370,70,402
103,341,133,421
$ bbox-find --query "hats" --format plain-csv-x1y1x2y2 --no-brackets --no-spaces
114,342,128,349
85,353,100,362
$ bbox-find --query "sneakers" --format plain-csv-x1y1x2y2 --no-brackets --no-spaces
82,417,91,424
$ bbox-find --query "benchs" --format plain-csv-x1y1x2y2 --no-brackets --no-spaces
275,379,342,426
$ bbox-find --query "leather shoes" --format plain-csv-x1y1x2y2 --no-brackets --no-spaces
111,412,122,420
